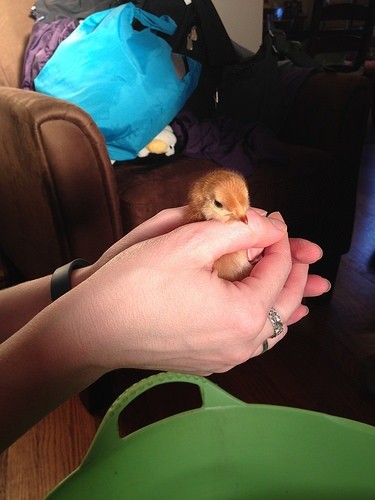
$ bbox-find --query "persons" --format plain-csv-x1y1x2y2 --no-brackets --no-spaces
0,203,333,455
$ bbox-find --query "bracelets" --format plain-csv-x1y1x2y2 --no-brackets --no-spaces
49,256,91,302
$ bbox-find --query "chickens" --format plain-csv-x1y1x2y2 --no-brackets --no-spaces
184,167,255,293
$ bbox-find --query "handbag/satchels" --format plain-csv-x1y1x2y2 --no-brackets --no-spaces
34,3,202,163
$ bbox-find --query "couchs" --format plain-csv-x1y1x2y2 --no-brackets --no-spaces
0,0,375,298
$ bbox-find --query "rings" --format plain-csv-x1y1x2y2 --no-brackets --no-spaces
263,339,270,354
267,305,284,339
